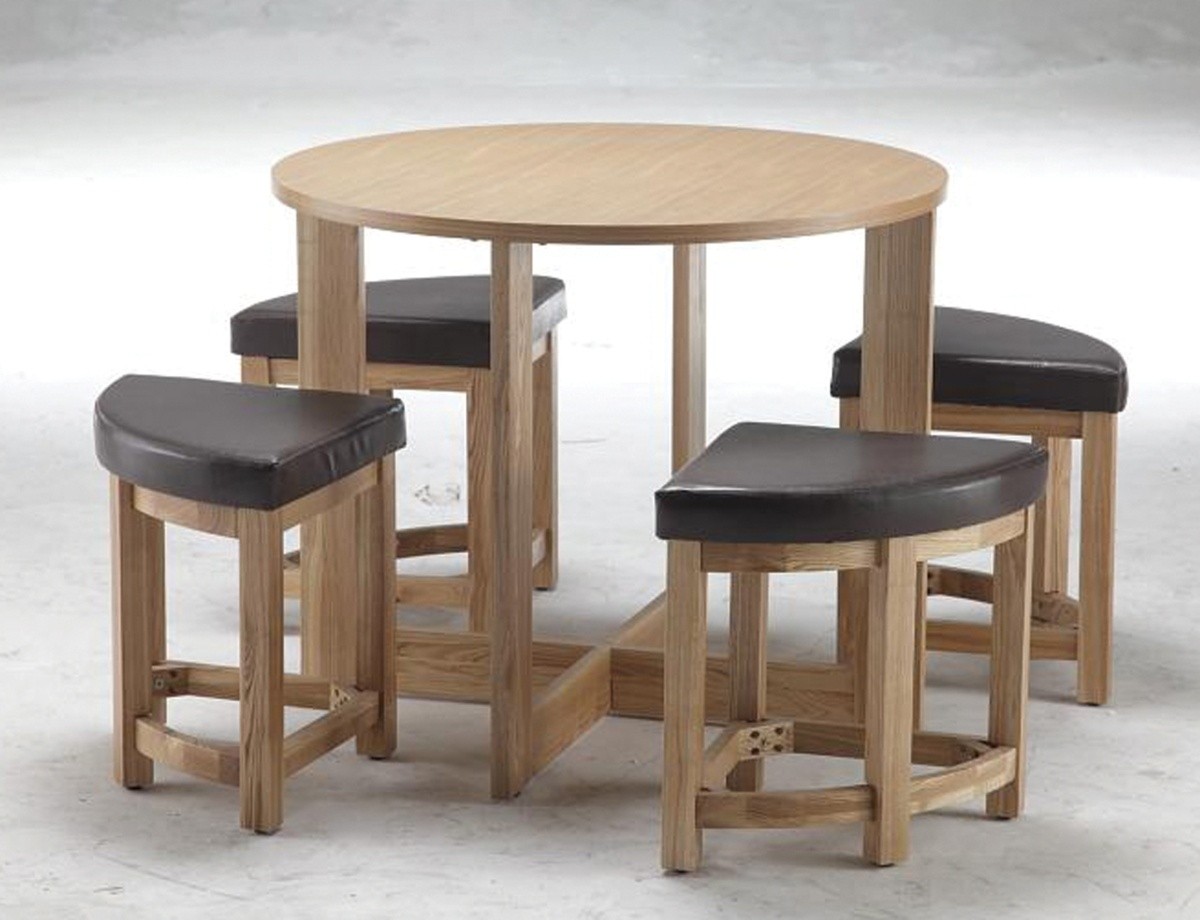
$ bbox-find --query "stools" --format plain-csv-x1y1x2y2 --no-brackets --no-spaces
231,275,566,636
830,304,1129,708
652,421,1050,871
94,374,409,834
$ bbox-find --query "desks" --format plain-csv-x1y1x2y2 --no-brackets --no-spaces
274,122,948,799
